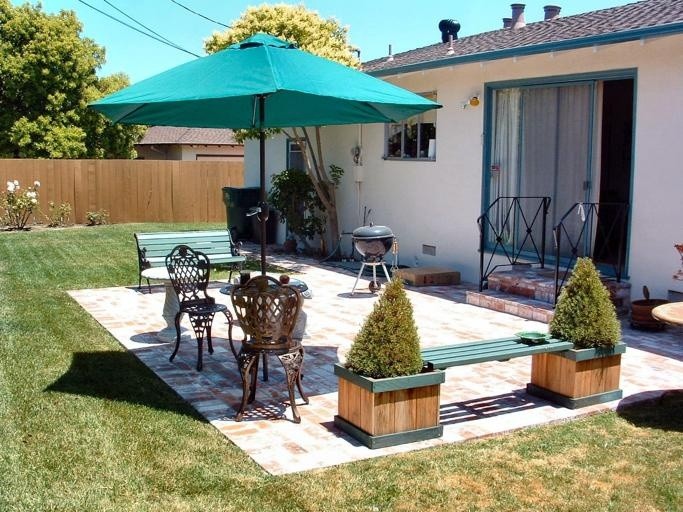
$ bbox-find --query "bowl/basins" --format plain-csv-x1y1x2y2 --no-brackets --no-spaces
513,331,552,346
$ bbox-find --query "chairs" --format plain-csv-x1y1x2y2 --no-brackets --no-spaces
164,245,238,372
230,277,308,423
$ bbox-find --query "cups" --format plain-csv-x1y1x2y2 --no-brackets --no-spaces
238,270,250,285
427,139,435,162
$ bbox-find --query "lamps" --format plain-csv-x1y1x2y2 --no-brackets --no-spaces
470,93,479,106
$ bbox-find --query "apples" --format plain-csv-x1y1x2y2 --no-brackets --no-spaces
279,275,289,284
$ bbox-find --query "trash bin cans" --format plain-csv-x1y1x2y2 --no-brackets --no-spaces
221,186,277,244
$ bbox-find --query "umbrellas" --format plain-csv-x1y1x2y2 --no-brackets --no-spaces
83,30,446,386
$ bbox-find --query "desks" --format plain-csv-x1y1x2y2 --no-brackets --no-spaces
650,302,682,407
139,264,203,343
219,277,308,382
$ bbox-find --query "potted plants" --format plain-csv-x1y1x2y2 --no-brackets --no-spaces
630,286,668,332
333,275,445,450
526,255,626,410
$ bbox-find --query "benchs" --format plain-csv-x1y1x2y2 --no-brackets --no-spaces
332,332,626,450
133,228,246,295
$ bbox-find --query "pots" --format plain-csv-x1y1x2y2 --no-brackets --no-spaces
352,223,393,259
